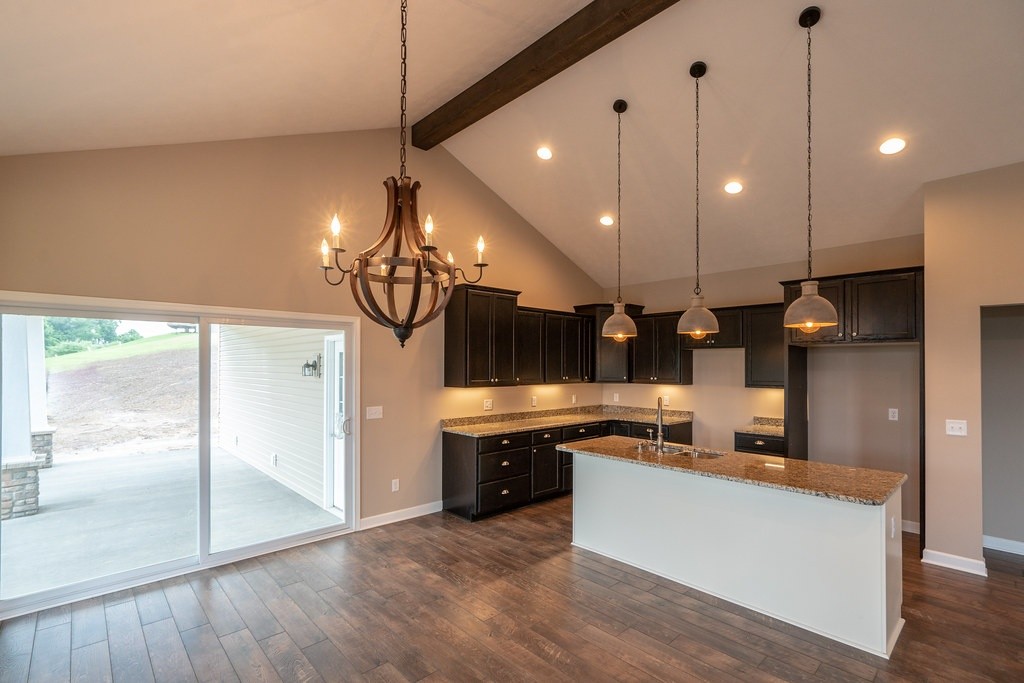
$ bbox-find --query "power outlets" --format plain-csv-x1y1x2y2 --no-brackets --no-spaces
484,399,492,410
572,395,577,404
946,420,967,436
664,396,669,406
392,479,399,492
891,516,896,538
366,406,384,419
614,393,619,402
889,409,898,421
531,397,536,407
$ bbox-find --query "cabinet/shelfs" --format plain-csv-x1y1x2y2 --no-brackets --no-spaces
780,266,923,345
735,431,784,457
590,303,631,384
746,301,783,389
443,283,523,389
545,310,590,386
632,311,681,385
681,305,743,349
443,422,693,522
518,308,545,386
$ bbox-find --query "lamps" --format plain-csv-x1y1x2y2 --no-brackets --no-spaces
783,5,840,335
302,353,321,379
318,0,489,349
677,61,720,340
602,98,637,344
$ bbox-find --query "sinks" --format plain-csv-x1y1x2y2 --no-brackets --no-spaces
634,438,686,455
672,448,726,460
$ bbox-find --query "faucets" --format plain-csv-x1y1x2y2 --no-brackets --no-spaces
650,397,665,455
